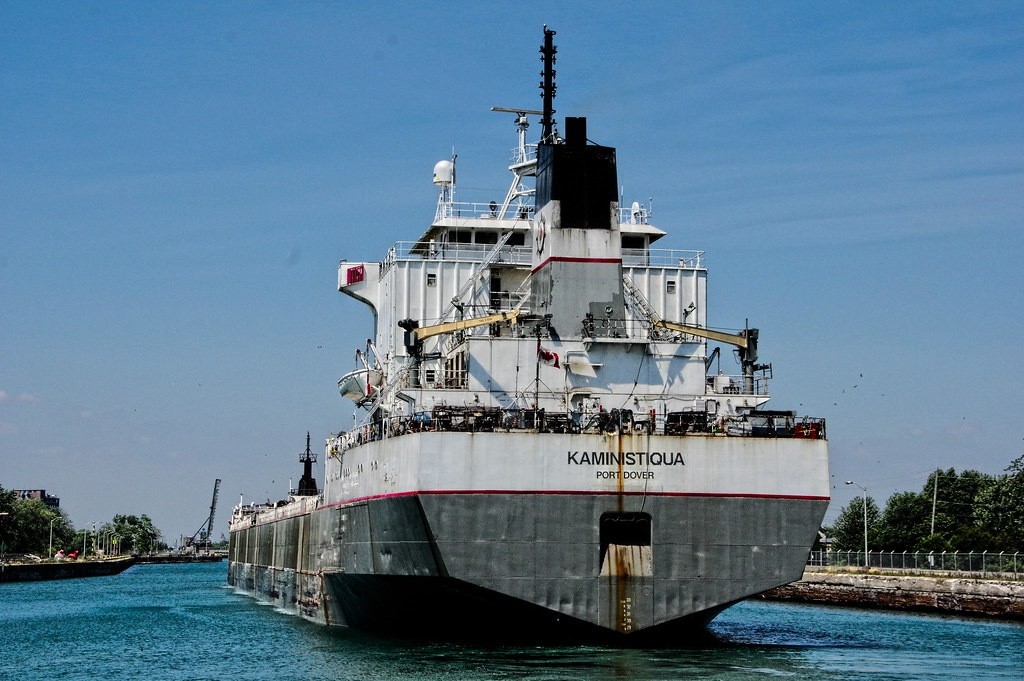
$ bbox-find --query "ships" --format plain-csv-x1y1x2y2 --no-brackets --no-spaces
222,24,833,638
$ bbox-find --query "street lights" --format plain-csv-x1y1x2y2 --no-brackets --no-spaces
81,519,124,557
49,515,61,561
846,480,871,571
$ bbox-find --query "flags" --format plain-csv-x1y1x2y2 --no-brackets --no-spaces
536,341,560,369
